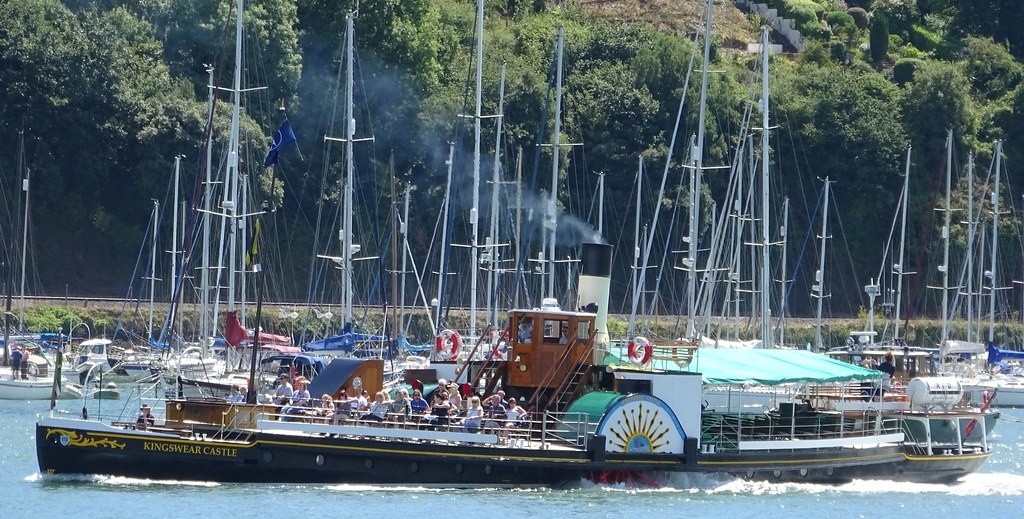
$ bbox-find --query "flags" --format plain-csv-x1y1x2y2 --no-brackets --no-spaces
264,118,295,169
246,218,261,266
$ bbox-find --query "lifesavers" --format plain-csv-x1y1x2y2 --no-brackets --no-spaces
436,329,458,359
627,336,652,365
492,330,510,360
441,330,463,361
671,337,694,368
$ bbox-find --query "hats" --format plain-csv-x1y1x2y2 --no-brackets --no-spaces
447,383,459,389
140,403,151,409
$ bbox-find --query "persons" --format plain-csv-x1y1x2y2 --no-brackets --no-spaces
861,355,896,402
11,346,29,381
225,372,528,446
137,404,155,431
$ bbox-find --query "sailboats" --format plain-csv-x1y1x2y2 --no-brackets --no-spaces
1,130,68,401
75,0,1024,407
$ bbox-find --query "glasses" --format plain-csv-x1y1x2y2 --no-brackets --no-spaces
414,395,420,396
472,401,478,403
279,378,283,380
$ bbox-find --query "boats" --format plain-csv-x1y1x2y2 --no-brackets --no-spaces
793,330,1001,445
763,376,992,482
36,164,904,482
